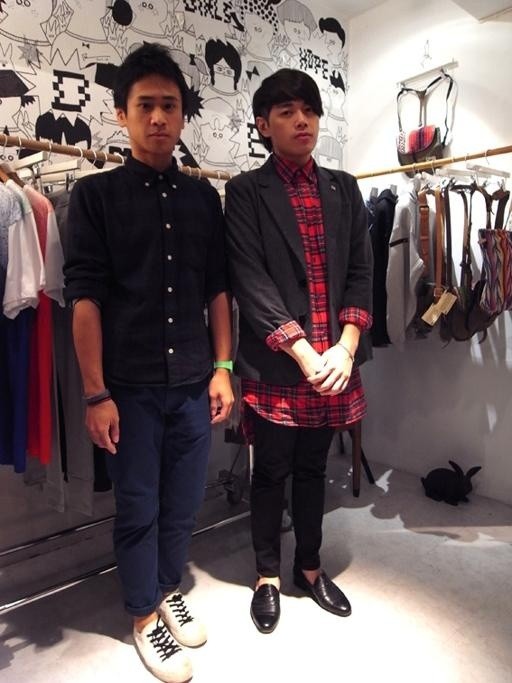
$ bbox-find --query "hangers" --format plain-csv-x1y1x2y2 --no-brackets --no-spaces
0,131,235,215
357,145,509,214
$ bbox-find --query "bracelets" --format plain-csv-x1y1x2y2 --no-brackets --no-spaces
84,391,111,405
213,361,235,373
340,344,356,363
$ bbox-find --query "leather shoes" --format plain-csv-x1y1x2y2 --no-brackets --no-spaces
250,576,281,634
293,563,352,617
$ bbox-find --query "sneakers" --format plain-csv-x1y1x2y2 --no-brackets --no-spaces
133,589,208,683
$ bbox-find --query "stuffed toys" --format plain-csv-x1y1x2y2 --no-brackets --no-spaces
421,459,481,505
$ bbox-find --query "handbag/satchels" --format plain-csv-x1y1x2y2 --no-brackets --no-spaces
414,228,512,341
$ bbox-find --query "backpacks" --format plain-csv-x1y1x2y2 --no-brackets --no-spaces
396,74,453,178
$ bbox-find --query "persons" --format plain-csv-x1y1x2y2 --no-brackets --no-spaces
62,45,248,683
224,69,376,634
